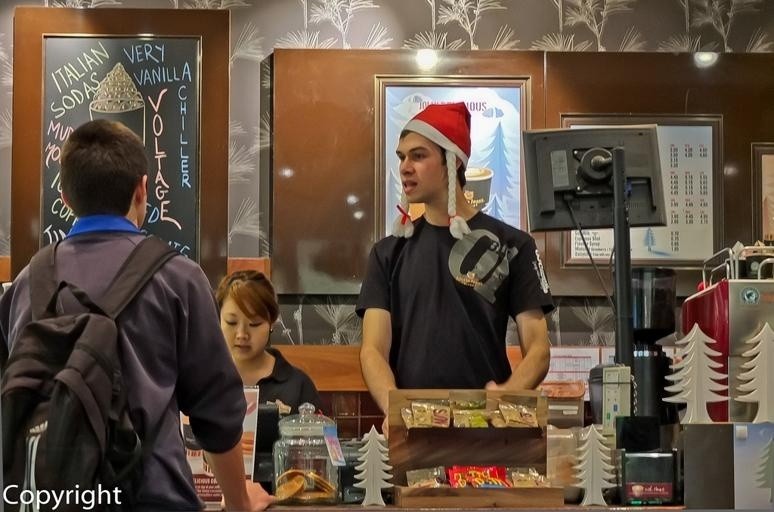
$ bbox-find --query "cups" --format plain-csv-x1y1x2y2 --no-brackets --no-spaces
462,166,495,212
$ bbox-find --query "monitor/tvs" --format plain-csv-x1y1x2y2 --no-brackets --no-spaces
523,123,667,234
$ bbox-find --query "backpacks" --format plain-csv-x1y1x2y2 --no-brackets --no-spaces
2,236,181,512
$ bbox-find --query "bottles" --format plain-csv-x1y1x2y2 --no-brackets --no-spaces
271,401,342,508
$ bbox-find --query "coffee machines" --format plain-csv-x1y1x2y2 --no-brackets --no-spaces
587,264,679,428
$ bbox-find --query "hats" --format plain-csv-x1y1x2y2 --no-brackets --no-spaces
403,102,471,169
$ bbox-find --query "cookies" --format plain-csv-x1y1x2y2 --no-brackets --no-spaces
275,469,338,505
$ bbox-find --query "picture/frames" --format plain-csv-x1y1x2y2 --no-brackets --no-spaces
371,72,536,254
556,109,728,271
749,142,774,246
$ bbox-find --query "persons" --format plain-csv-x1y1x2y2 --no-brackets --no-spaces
217,269,331,494
1,118,279,510
353,99,557,441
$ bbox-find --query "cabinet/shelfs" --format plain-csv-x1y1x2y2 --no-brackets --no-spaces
266,344,385,440
